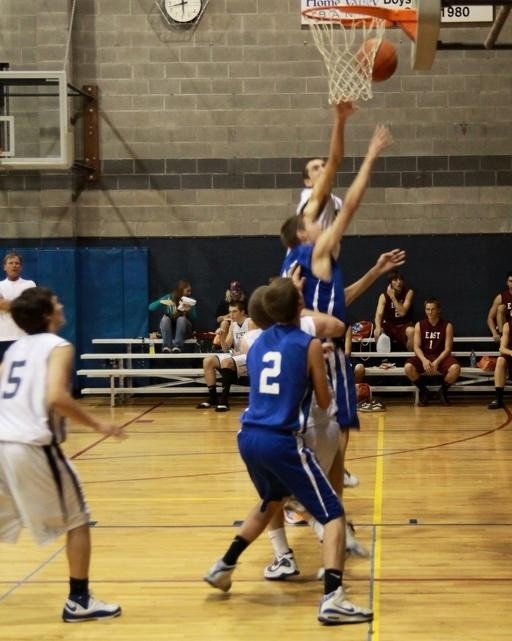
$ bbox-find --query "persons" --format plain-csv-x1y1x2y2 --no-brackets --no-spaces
0,287,130,623
197,413,408,629
1,253,37,362
277,102,407,264
147,265,512,416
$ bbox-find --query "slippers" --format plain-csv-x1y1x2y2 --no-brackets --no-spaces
196,402,230,412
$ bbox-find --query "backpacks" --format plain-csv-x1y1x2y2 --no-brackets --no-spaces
351,320,374,342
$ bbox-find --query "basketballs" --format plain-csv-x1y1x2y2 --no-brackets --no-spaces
355,38,399,82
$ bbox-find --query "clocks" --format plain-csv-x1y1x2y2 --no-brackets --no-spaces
164,0,202,23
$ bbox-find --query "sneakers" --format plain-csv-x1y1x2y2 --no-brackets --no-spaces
63,598,121,623
318,586,373,622
418,391,450,407
488,400,505,409
203,558,235,592
356,399,386,411
264,469,358,579
162,347,181,353
